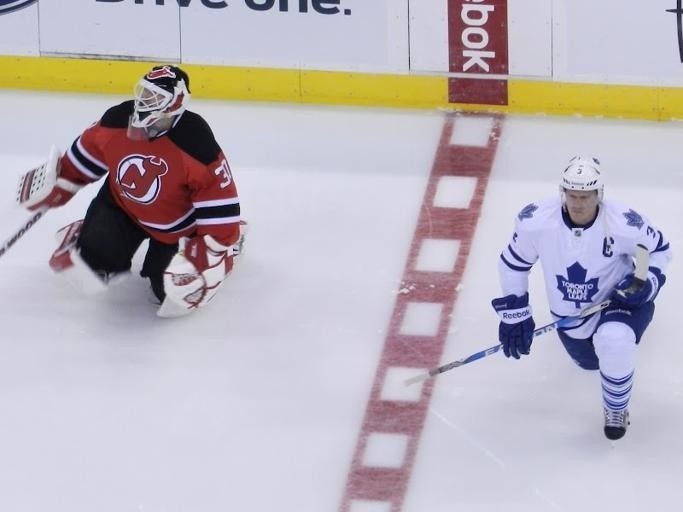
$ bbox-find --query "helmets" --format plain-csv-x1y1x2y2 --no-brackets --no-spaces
561,154,603,197
128,65,192,139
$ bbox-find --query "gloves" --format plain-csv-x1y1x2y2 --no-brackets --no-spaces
611,267,665,310
492,292,536,359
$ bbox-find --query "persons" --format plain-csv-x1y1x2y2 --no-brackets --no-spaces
492,153,674,439
12,65,243,312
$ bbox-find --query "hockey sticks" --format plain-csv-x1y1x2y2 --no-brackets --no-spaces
404,244,650,385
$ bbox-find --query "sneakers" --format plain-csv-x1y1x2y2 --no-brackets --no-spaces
604,407,626,439
